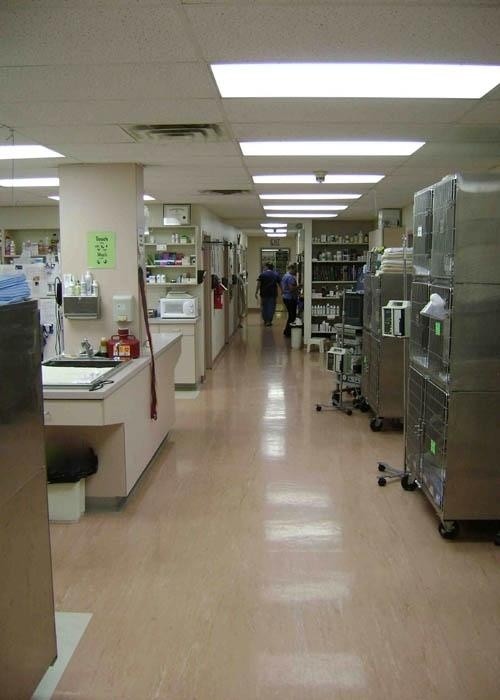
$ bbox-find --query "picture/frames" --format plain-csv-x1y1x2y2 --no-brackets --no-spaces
162,202,193,226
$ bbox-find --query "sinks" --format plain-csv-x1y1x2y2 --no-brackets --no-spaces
41,359,134,388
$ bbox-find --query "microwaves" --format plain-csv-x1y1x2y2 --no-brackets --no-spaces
160,297,199,318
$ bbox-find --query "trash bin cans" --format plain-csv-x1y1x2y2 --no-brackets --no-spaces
47,477,86,523
290,323,303,348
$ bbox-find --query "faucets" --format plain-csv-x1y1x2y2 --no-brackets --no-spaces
81,337,96,356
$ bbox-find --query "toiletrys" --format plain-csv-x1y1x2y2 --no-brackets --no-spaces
64,271,99,297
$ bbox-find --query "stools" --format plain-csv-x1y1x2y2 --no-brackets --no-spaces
306,337,329,351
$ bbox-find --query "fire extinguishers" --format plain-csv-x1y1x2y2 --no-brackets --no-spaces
214,278,223,310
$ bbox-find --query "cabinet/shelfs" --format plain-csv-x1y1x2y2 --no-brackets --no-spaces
43,400,102,430
361,270,410,420
313,241,367,334
150,324,200,384
404,172,500,524
143,225,200,286
2,228,59,268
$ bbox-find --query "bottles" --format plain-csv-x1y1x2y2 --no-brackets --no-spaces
351,265,357,281
322,321,331,331
171,233,179,243
100,337,107,353
69,271,99,297
312,303,340,315
312,229,368,243
10,241,16,256
317,247,368,261
161,272,166,283
156,304,160,317
157,273,161,283
51,233,57,248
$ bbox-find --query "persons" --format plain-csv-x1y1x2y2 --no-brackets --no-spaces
255,262,282,326
281,261,303,337
